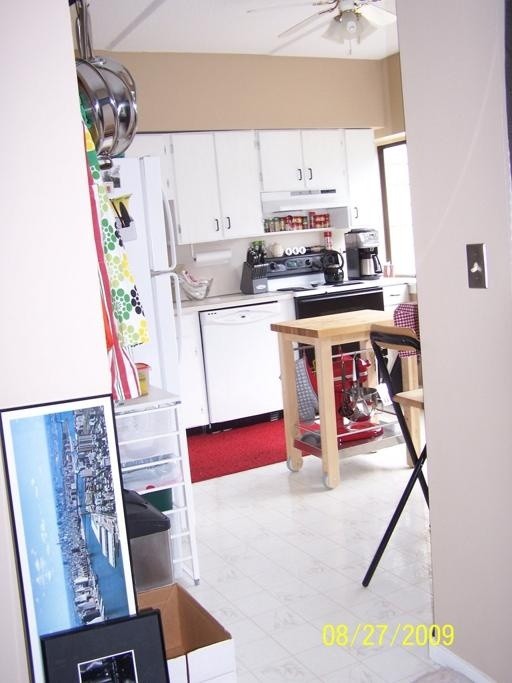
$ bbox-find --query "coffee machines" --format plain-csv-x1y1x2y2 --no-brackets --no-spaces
344,228,383,279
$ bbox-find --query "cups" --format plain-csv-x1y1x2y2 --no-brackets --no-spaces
384,264,393,277
135,362,151,395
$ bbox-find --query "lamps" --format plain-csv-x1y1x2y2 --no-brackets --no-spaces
321,11,382,44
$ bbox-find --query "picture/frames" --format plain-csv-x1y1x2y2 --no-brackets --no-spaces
39,609,170,683
0,394,138,683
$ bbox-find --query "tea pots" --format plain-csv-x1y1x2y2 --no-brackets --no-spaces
320,251,344,282
270,241,283,257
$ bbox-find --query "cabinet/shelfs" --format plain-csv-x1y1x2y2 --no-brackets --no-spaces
102,133,180,245
115,384,201,586
170,130,264,245
344,129,386,275
257,129,348,214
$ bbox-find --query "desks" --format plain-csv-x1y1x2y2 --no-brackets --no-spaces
270,310,421,489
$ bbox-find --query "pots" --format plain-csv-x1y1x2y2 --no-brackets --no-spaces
73,1,140,169
336,386,382,423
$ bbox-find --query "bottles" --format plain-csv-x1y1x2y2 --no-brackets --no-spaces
251,241,267,258
323,231,332,249
265,211,330,232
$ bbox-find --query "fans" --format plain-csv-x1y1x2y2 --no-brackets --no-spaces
246,0,397,55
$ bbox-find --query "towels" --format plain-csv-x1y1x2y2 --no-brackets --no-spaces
279,359,319,422
393,301,420,358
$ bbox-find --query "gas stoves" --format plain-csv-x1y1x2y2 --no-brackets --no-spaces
273,280,378,296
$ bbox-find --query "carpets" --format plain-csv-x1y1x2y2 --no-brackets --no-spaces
187,410,384,484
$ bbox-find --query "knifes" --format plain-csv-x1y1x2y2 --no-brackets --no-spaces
248,246,266,265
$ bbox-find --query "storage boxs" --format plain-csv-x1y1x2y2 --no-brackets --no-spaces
136,581,238,683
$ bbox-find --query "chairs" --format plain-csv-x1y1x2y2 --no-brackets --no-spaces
362,323,429,586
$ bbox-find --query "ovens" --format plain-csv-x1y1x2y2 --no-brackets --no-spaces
294,288,383,370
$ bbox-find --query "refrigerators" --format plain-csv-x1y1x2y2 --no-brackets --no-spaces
100,152,182,395
